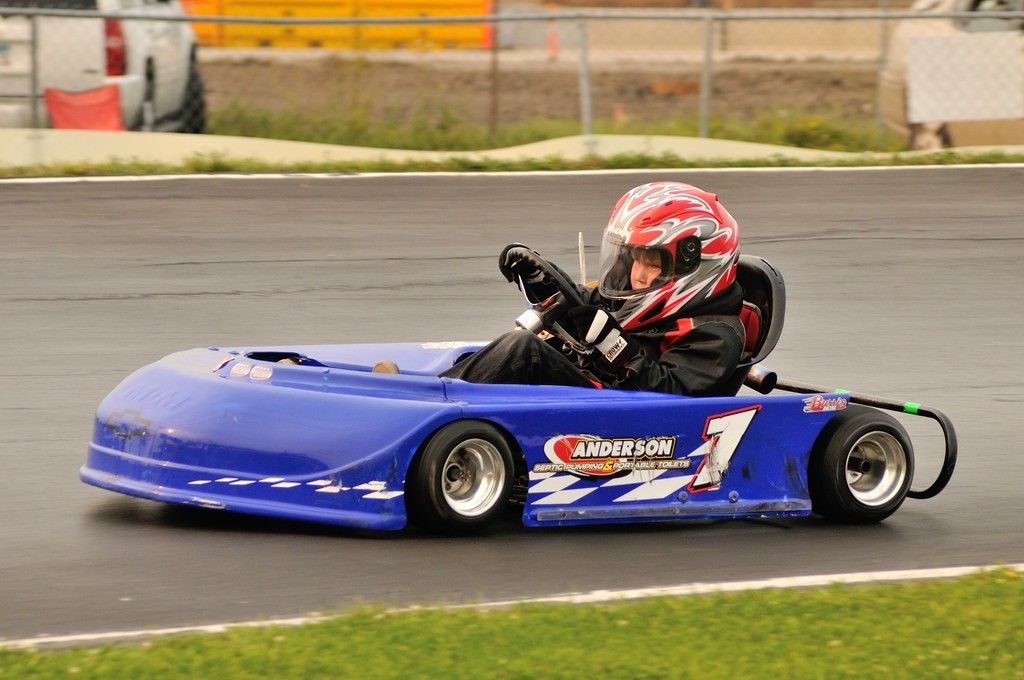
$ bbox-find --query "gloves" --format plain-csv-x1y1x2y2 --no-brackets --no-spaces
559,303,640,374
498,241,542,282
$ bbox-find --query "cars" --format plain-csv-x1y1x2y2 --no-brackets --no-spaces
873,0,1024,152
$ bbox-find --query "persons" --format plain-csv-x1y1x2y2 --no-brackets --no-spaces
372,181,747,396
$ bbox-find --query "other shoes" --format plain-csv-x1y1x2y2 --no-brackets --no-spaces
372,360,399,374
277,357,301,365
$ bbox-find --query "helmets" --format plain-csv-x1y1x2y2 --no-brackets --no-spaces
597,180,741,334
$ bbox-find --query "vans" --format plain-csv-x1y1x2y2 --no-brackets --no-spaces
0,1,206,137
